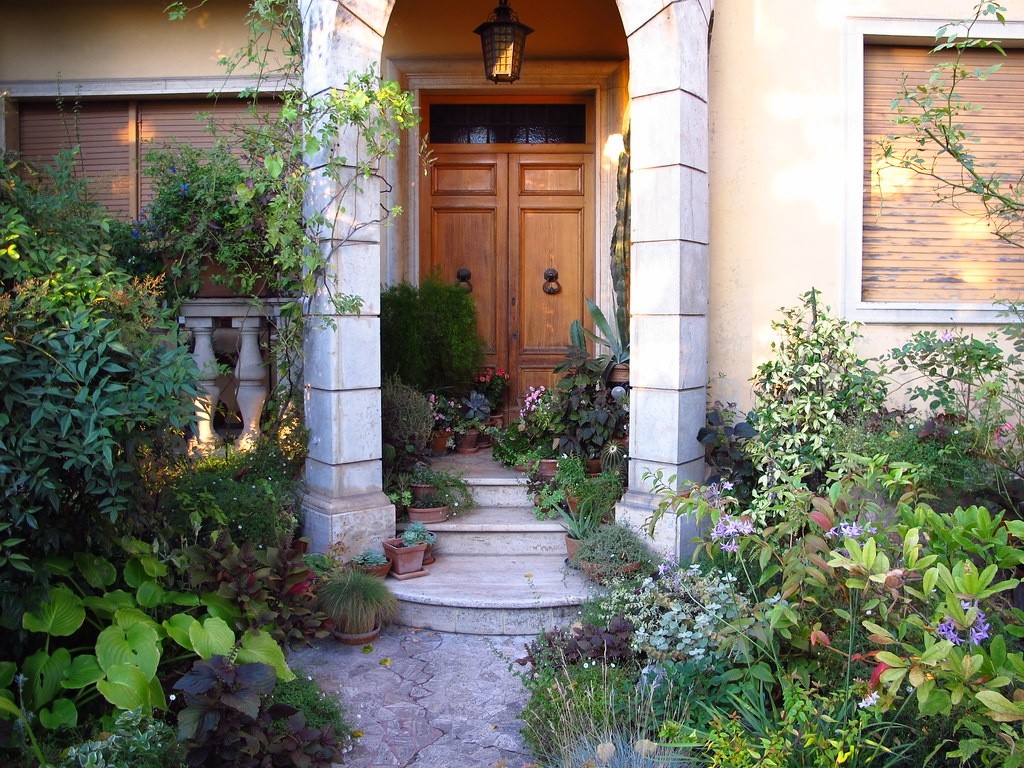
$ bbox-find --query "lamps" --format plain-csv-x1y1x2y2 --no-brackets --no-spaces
472,0,534,85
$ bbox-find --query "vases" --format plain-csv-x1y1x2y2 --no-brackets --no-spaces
490,412,503,431
426,430,452,457
160,259,275,298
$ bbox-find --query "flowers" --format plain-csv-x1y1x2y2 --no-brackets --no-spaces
131,144,300,270
520,385,558,430
474,368,510,415
426,393,462,432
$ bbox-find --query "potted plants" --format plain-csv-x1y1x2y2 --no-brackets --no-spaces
382,468,413,523
538,490,603,571
528,438,559,476
445,417,485,454
411,464,438,500
407,492,450,525
461,390,497,449
493,423,532,473
554,452,581,512
567,523,660,586
319,572,397,643
382,532,429,581
550,344,626,476
350,547,392,580
397,522,436,565
